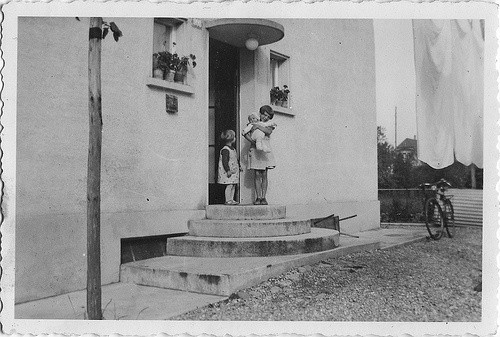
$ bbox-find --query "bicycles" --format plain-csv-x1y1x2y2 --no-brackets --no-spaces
419,179,456,241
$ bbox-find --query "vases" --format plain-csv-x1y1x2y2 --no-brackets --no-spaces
165,70,174,81
276,100,282,106
174,71,185,84
281,101,287,107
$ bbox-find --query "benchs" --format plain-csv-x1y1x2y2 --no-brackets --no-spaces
311,213,361,239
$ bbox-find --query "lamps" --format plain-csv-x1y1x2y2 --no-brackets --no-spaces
246,34,259,50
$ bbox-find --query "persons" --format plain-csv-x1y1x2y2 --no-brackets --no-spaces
217,130,244,205
242,114,277,153
246,104,275,205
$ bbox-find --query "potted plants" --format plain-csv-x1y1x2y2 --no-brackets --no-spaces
270,87,278,105
152,50,173,80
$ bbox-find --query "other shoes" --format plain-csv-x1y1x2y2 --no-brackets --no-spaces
225,200,239,204
261,198,268,205
254,198,261,205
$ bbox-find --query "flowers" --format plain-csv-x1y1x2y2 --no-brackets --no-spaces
276,87,284,100
164,40,180,70
281,85,290,101
175,54,197,72
